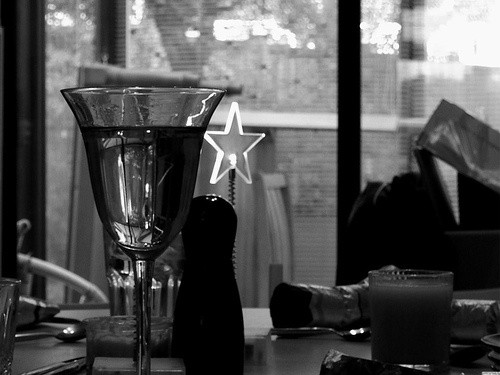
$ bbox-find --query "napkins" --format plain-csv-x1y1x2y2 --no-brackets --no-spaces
451,298,500,339
270,264,400,329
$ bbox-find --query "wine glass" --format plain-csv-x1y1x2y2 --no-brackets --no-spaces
59,85,227,374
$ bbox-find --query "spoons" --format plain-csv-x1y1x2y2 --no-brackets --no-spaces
14,322,86,343
270,326,370,342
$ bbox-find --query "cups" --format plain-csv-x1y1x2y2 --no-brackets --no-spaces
85,315,187,375
368,270,453,375
102,225,183,316
0,278,21,375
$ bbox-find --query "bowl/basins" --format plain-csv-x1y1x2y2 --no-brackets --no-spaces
481,334,500,358
449,341,491,367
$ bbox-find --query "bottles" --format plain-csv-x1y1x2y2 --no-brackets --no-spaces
171,194,246,375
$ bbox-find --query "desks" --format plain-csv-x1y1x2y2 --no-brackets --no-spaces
9,288,500,375
